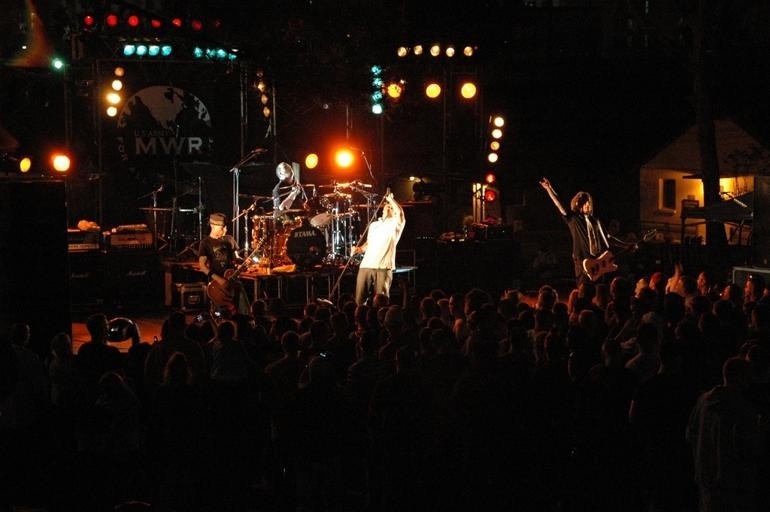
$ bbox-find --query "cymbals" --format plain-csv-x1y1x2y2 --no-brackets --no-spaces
280,208,303,214
239,194,272,200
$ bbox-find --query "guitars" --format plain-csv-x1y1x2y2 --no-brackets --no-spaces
207,236,267,306
583,229,656,282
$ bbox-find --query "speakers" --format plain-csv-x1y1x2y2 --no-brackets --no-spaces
733,266,770,291
0,177,72,358
68,248,153,315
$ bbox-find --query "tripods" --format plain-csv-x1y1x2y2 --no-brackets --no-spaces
333,200,345,246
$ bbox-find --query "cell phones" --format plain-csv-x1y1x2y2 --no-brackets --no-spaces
196,315,204,323
215,311,221,317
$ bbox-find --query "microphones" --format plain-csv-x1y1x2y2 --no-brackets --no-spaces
255,148,268,154
719,191,729,195
387,188,391,203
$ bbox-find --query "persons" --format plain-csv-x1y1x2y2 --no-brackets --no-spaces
350,193,407,313
197,211,253,317
539,177,639,289
272,160,308,217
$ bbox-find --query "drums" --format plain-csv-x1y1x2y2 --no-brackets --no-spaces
305,196,332,227
329,196,354,217
272,226,326,267
252,218,280,251
